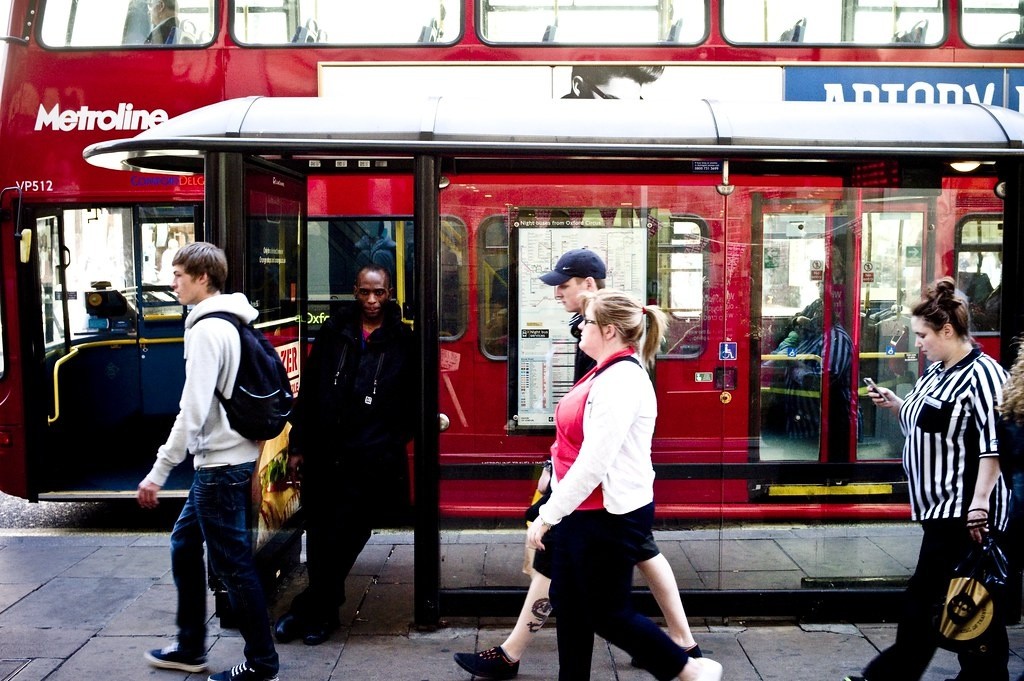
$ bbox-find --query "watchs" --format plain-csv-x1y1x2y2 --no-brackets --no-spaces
543,460,552,471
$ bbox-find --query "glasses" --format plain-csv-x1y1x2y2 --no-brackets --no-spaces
584,315,616,337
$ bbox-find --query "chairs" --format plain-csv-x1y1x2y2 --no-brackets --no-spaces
163,18,1024,44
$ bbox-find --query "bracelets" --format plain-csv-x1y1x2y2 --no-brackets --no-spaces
540,518,552,530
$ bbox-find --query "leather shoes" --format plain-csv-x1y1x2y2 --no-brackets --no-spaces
274,592,312,645
303,610,341,647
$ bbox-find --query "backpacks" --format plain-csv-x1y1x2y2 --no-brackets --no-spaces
189,312,291,441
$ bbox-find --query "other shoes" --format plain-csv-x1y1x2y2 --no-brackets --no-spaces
843,675,869,681
691,656,723,681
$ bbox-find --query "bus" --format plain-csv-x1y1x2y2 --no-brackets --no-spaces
0,0,1023,530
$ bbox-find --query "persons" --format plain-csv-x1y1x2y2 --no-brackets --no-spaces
786,294,864,460
142,0,180,45
455,252,722,681
136,243,280,681
844,278,1024,681
761,272,827,368
273,261,414,647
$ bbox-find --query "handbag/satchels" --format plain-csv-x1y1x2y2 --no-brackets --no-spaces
930,532,1012,656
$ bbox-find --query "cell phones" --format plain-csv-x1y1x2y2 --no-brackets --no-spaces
864,378,889,402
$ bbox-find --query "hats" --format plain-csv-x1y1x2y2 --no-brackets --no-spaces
540,255,606,286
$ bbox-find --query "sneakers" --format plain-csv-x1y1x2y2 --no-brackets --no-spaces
207,661,279,681
453,646,520,680
630,644,702,668
144,644,210,672
